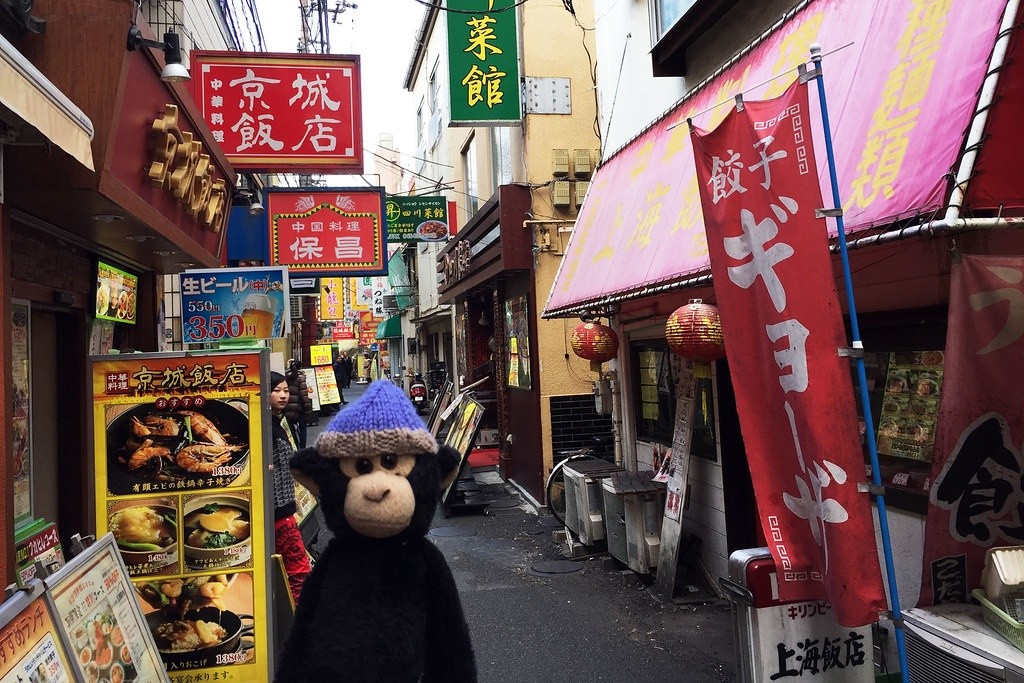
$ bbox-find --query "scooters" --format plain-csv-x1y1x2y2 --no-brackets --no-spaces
408,371,431,414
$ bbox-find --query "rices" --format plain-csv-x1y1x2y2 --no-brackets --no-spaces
154,620,227,653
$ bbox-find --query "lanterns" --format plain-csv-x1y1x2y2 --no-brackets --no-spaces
666,298,727,379
570,320,620,371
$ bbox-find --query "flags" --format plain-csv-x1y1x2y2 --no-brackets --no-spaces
684,79,891,629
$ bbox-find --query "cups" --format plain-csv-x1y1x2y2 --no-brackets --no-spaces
239,294,278,338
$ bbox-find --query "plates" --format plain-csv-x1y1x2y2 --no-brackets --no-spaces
97,286,109,315
118,292,129,318
81,620,133,683
127,294,136,319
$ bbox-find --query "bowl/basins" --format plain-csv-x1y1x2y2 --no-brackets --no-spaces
146,609,256,670
105,400,250,492
108,504,179,568
184,495,252,568
417,220,448,240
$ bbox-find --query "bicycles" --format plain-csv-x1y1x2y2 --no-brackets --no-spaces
545,432,618,525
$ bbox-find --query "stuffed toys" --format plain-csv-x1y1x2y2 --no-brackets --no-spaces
271,380,478,683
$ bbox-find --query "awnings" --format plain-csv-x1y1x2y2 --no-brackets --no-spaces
542,0,1024,322
375,317,401,340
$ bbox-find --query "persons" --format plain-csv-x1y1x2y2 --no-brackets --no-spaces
285,360,311,450
269,371,312,608
333,355,349,405
341,351,352,389
363,354,372,388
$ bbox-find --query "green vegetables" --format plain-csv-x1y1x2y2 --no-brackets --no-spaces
114,514,177,551
203,502,238,548
